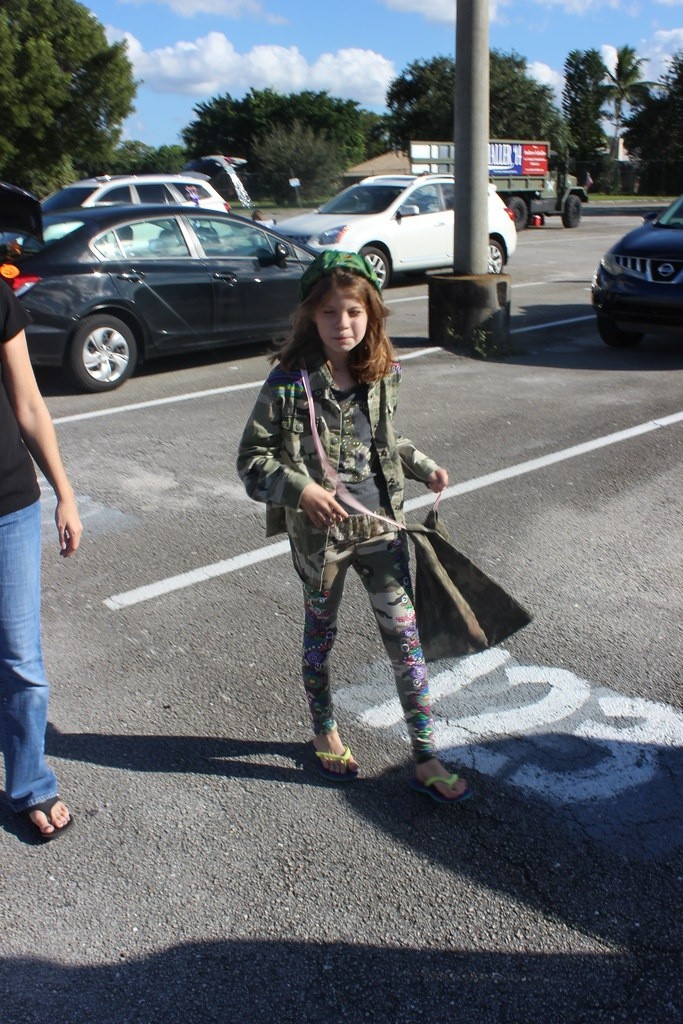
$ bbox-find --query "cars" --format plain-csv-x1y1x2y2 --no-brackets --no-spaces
590,193,683,350
0,183,329,394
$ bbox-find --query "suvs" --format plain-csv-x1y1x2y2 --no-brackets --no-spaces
38,171,232,255
270,172,517,291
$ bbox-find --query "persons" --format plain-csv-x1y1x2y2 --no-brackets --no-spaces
0,279,85,844
232,248,476,806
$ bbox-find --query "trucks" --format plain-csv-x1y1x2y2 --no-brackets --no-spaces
407,138,590,232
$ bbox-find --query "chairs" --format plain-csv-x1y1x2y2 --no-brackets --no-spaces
154,230,189,256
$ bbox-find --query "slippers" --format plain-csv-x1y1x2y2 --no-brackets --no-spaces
408,772,474,805
310,739,361,781
17,798,74,839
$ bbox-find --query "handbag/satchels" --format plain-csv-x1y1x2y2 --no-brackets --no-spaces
404,508,534,664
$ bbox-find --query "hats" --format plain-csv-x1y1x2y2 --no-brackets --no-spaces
300,249,383,301
